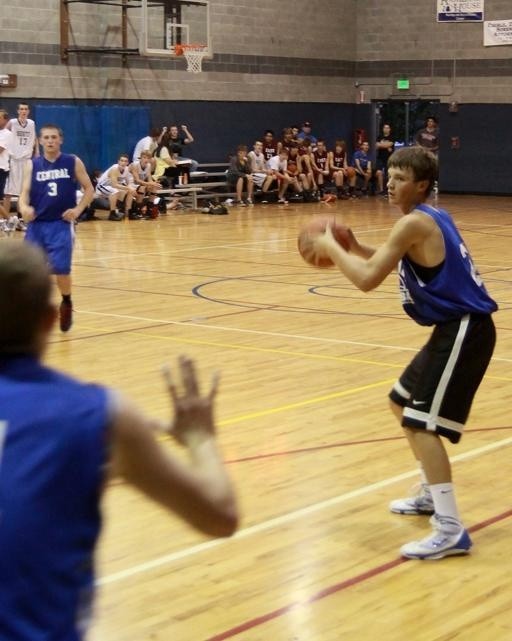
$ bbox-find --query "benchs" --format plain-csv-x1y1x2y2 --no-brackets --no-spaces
11,162,360,210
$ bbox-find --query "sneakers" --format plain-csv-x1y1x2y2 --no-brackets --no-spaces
247,199,254,206
398,515,474,559
237,201,246,207
0,216,27,231
59,301,72,332
389,481,436,516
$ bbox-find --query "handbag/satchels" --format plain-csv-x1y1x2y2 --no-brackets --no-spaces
201,201,229,215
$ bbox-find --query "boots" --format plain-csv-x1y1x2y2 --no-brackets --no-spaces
110,209,121,220
129,210,141,219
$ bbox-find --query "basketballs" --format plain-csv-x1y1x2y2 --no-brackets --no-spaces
346,167,355,178
298,218,351,267
288,165,298,173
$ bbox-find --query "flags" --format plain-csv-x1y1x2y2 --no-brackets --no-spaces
483,19,512,48
436,0,484,23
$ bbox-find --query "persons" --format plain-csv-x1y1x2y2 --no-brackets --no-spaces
1,102,36,232
97,123,196,222
301,143,496,560
21,124,94,332
0,109,18,233
228,121,399,209
1,236,240,640
413,116,441,194
